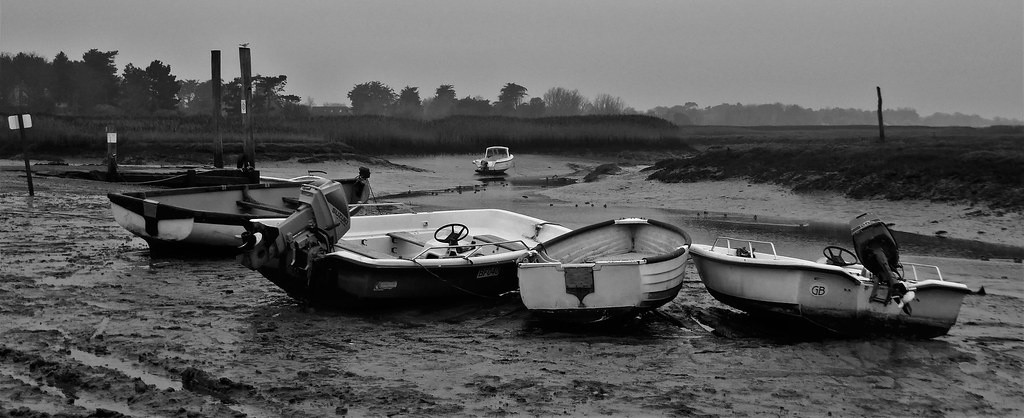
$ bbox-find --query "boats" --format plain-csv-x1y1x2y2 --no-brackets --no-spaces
689,211,971,338
234,177,573,309
473,146,514,174
107,166,371,257
515,217,691,327
